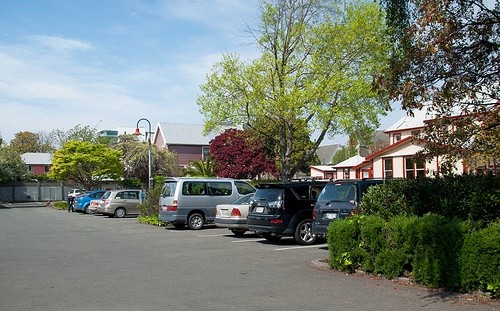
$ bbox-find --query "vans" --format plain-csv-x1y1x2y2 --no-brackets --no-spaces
96,189,146,218
158,177,257,230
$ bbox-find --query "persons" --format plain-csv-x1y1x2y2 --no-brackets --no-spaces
67,196,75,213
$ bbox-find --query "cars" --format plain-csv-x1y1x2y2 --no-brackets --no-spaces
68,187,107,214
214,193,256,235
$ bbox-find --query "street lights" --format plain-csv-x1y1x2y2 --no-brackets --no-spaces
133,118,153,191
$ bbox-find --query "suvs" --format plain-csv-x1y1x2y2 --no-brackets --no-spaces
311,177,408,235
246,178,333,245
